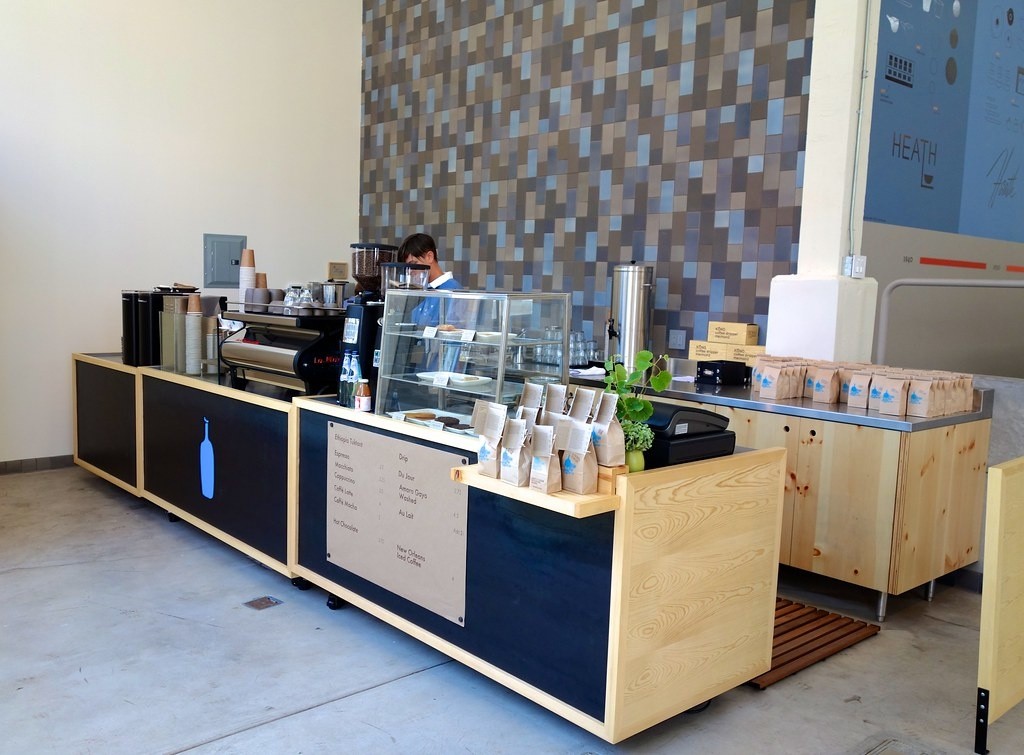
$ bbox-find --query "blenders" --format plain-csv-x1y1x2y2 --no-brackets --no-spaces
346,244,399,305
367,263,432,408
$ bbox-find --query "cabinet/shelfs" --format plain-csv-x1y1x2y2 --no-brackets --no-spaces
570,373,996,624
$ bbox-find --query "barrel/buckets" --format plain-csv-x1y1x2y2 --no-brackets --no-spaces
608,265,657,369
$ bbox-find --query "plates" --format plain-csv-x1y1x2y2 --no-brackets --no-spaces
477,332,517,339
417,372,491,386
439,330,463,334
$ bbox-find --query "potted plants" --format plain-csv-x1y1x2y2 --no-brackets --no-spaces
621,420,655,472
605,350,671,473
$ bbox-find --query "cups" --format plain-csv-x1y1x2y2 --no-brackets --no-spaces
532,326,597,364
239,249,339,316
186,294,202,375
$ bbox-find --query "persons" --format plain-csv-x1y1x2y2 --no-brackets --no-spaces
397,233,469,408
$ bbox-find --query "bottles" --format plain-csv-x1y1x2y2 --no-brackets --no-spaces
354,379,371,412
340,349,352,406
347,351,362,407
